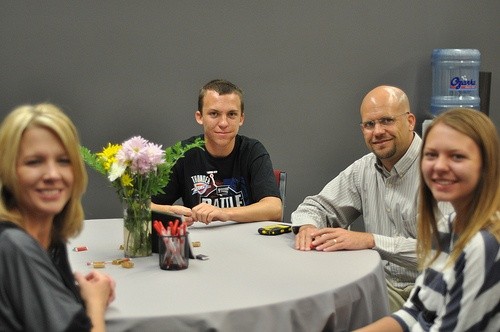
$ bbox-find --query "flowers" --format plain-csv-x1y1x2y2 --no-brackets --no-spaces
80,134,207,256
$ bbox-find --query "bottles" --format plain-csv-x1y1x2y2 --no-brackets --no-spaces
431,49,480,114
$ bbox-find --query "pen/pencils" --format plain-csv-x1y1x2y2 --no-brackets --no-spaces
87,261,113,266
153,220,186,269
122,258,132,261
74,246,88,251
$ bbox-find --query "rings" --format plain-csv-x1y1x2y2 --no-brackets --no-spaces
333,238,337,244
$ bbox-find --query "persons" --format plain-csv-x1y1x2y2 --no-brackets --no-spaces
290,85,456,315
347,108,500,332
0,103,116,332
141,79,283,225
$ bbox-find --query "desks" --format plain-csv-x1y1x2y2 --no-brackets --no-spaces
66,219,391,332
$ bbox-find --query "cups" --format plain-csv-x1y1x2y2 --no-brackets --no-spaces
159,232,189,270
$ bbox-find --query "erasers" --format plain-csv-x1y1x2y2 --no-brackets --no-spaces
196,254,207,260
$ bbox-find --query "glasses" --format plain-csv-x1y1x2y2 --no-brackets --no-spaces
358,111,409,129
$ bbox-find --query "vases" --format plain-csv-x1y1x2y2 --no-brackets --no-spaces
123,198,153,259
